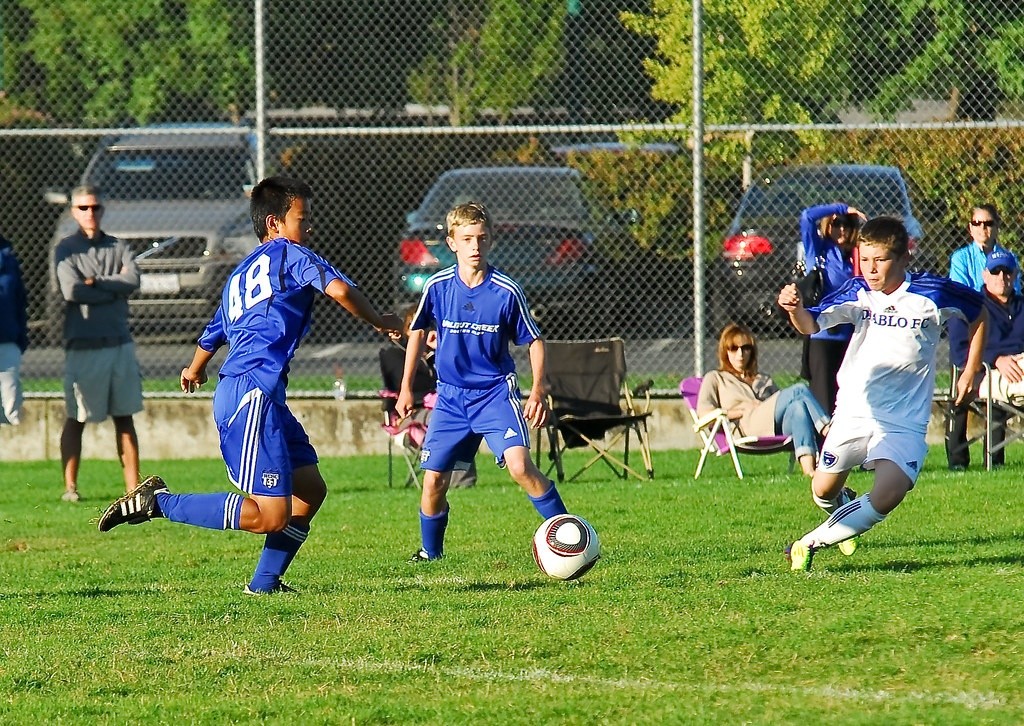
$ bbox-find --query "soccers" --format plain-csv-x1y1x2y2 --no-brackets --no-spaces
530,514,602,582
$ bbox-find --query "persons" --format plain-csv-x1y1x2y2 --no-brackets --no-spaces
696,323,831,479
777,214,989,570
379,301,481,489
939,202,1024,471
0,233,31,425
55,185,143,502
99,176,404,598
799,202,876,468
394,202,568,561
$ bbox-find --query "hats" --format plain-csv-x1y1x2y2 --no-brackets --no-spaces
985,252,1017,271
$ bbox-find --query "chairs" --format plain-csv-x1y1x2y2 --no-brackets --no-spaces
520,337,656,483
680,377,794,480
932,362,1024,472
379,347,437,492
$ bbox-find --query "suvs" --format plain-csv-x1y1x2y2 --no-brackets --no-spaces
42,124,279,349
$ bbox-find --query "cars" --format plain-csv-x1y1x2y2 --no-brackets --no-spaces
713,160,926,336
394,165,638,338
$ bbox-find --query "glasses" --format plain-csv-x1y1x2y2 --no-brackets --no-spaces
73,204,103,212
971,220,995,227
988,265,1014,275
725,343,752,351
828,218,851,228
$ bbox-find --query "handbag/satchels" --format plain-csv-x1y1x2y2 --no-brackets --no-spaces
788,239,825,314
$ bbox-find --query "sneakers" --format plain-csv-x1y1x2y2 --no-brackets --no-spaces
239,581,300,596
784,540,812,574
61,491,79,502
835,488,860,555
404,547,430,566
99,475,171,533
